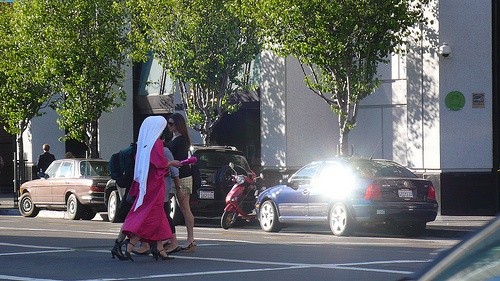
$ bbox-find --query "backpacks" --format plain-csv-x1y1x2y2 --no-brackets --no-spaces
109,147,135,188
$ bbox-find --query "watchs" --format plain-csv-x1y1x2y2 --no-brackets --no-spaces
176,185,182,192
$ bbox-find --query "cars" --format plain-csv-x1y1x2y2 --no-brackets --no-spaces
255,154,440,237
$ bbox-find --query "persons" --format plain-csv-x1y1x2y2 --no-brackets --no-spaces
111,113,198,262
36,144,57,173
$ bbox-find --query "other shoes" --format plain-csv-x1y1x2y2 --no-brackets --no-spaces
163,242,171,247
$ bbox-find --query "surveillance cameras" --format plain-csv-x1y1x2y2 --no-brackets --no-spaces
440,45,451,57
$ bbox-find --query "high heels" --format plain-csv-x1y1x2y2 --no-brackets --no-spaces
119,238,135,263
131,249,151,256
153,248,175,261
181,240,197,253
165,246,182,256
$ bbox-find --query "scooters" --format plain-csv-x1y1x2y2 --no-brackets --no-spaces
220,161,289,229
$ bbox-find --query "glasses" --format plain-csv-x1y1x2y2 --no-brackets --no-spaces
167,122,175,126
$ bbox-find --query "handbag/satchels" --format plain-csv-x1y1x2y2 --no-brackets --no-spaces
190,165,201,190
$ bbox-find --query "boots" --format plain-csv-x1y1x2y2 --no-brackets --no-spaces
111,239,129,261
149,244,156,255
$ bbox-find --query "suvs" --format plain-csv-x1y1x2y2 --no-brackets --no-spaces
104,142,258,227
17,157,111,221
